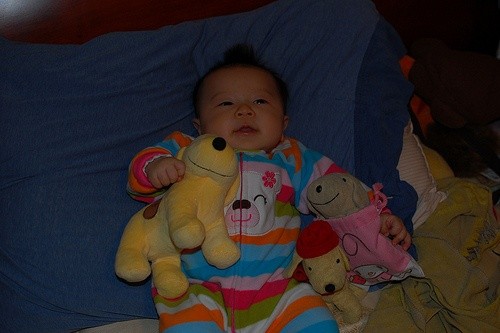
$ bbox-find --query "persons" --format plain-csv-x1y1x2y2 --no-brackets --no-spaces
124,42,412,333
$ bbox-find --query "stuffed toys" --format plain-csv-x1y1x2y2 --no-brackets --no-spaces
281,220,376,324
115,133,242,300
307,172,426,292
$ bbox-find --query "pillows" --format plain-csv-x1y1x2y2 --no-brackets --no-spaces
0,1,448,333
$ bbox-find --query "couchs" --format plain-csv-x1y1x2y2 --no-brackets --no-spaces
1,1,500,333
